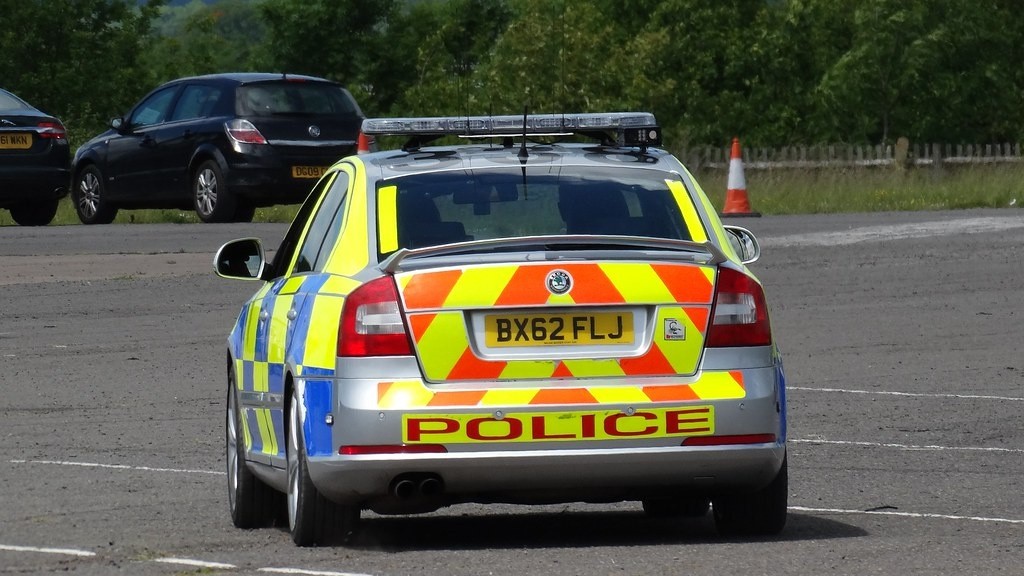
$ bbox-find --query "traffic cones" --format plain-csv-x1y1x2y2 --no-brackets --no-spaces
716,136,766,219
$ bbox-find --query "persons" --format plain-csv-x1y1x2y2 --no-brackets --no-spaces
397,190,442,224
558,183,618,235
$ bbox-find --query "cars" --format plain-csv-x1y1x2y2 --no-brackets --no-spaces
0,90,74,228
212,110,790,548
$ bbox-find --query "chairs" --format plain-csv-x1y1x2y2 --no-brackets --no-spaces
396,196,471,247
559,184,631,232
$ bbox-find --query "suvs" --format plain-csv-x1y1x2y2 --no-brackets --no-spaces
72,72,370,224
